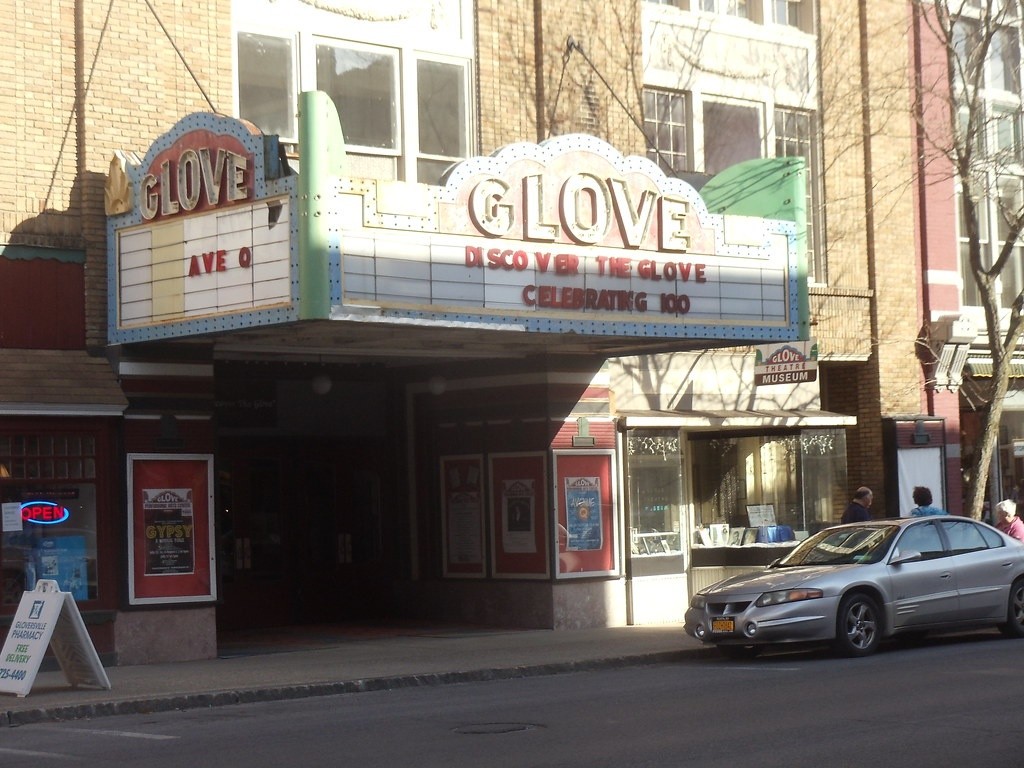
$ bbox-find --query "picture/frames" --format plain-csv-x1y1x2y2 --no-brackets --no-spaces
697,503,777,547
998,425,1012,497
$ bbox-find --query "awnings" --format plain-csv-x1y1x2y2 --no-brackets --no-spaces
961,349,1024,381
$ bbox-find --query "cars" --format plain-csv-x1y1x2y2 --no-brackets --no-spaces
683,515,1024,658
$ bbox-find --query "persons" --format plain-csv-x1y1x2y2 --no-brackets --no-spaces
840,486,874,525
905,485,948,517
994,499,1024,545
